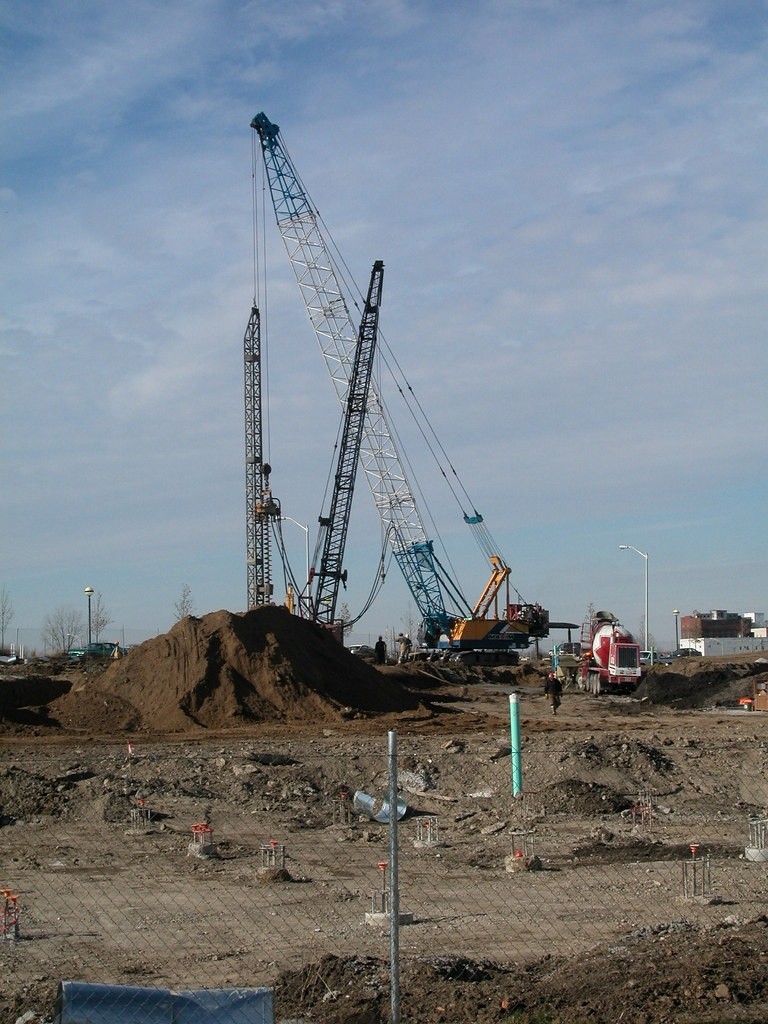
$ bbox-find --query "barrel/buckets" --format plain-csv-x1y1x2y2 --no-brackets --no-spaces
25,659,29,664
744,704,751,711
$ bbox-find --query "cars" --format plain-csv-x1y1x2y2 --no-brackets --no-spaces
519,642,589,663
672,648,704,659
0,651,81,668
348,644,375,658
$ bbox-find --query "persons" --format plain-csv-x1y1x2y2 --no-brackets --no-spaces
375,636,387,665
395,633,412,663
544,673,563,715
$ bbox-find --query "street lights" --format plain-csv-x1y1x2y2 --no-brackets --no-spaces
673,609,680,650
85,586,95,643
619,545,649,651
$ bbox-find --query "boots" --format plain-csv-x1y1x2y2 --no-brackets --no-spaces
551,705,556,715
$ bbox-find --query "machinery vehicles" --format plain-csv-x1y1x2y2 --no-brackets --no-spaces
244,298,282,614
291,259,387,641
247,108,550,674
576,612,641,697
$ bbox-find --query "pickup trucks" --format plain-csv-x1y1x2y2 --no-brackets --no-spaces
639,649,677,667
64,641,127,664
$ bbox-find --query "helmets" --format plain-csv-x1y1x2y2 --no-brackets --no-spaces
549,674,554,678
